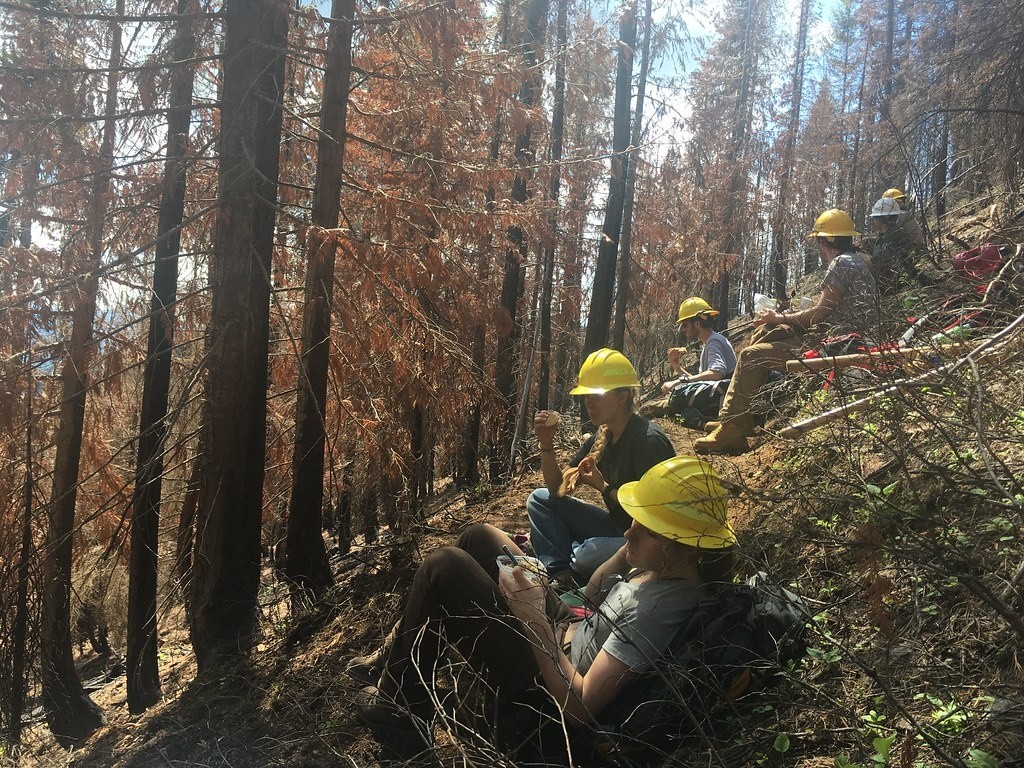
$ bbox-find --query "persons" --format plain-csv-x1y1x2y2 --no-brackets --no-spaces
696,207,882,454
850,189,926,296
346,454,736,764
640,296,736,419
526,349,676,594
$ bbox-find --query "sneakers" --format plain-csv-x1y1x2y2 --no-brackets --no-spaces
692,414,755,454
343,636,439,748
546,565,578,591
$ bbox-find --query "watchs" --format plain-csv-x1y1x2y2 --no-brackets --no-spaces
679,374,688,383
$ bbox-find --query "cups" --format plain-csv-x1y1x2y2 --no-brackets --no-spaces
799,295,816,310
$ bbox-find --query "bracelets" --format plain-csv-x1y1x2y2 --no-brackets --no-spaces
539,443,554,452
601,482,614,497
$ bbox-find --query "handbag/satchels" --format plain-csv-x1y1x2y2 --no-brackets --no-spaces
668,371,790,430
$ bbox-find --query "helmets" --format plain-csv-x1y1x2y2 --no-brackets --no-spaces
568,347,644,396
806,208,863,239
881,187,907,201
615,454,738,550
868,198,906,217
675,296,719,325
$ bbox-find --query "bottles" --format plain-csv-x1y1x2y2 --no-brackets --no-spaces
952,243,1010,276
